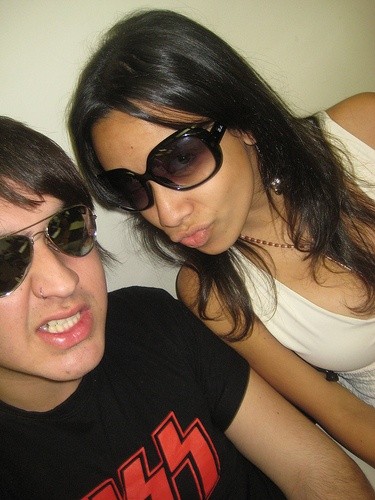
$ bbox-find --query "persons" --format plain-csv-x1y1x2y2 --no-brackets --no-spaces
0,113,375,500
66,10,375,492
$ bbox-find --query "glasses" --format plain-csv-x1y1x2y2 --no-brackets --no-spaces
96,117,233,212
0,205,103,298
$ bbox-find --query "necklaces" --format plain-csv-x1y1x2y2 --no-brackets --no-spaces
238,232,352,272
